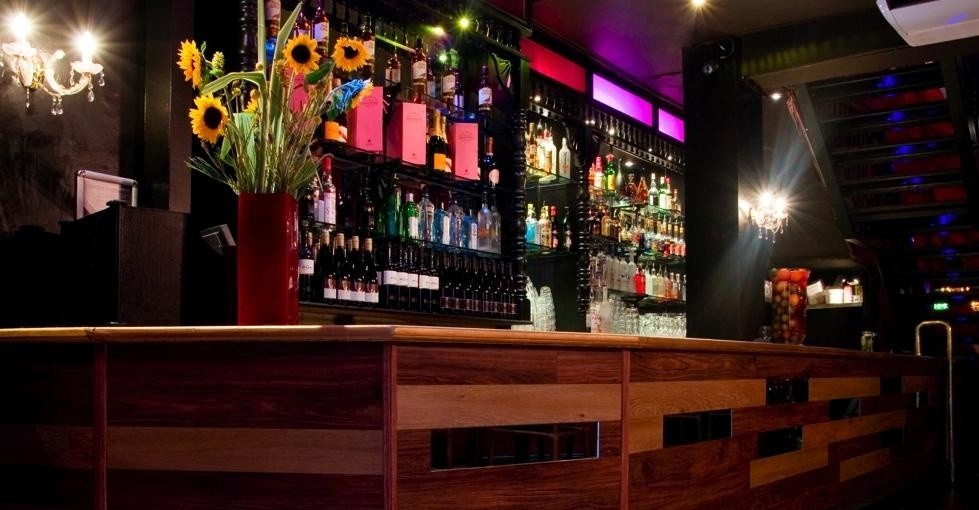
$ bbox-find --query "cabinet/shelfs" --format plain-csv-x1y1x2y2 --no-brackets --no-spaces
248,0,694,334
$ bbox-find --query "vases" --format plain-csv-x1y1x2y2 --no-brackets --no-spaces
235,193,298,325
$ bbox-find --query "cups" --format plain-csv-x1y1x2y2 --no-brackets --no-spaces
615,298,687,338
526,281,555,332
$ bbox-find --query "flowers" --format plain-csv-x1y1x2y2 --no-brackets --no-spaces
178,0,375,191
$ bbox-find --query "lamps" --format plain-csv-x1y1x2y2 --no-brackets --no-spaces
1,12,109,117
741,193,792,242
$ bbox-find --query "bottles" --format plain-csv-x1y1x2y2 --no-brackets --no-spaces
593,199,686,257
843,286,853,304
525,201,572,254
863,332,873,352
591,151,683,214
528,118,572,185
290,1,499,190
301,158,503,254
263,0,283,78
598,286,613,335
298,226,517,316
595,246,687,302
